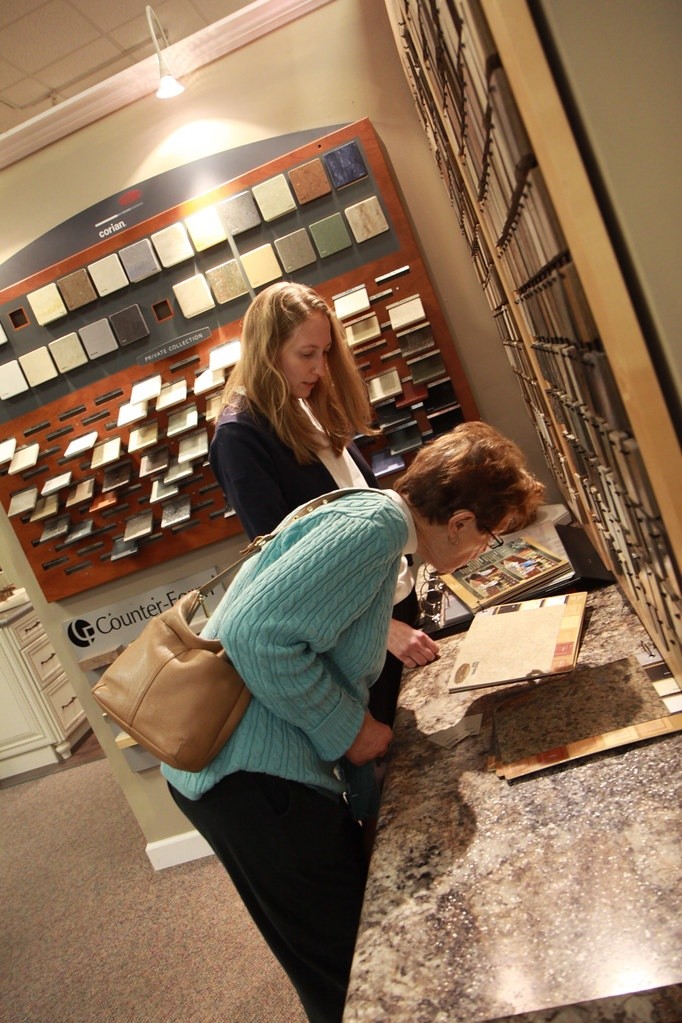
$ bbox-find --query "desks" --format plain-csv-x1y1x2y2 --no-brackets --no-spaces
342,519,682,1023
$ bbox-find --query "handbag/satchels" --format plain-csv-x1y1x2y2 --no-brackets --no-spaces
91,590,253,774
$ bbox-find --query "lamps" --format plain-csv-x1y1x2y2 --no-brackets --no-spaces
145,5,185,99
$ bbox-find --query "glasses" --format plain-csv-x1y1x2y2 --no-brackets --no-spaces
486,529,504,549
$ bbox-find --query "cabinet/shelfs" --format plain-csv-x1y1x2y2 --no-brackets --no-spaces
0,610,91,783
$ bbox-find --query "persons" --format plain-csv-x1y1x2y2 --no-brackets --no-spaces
208,281,440,726
158,421,547,1023
